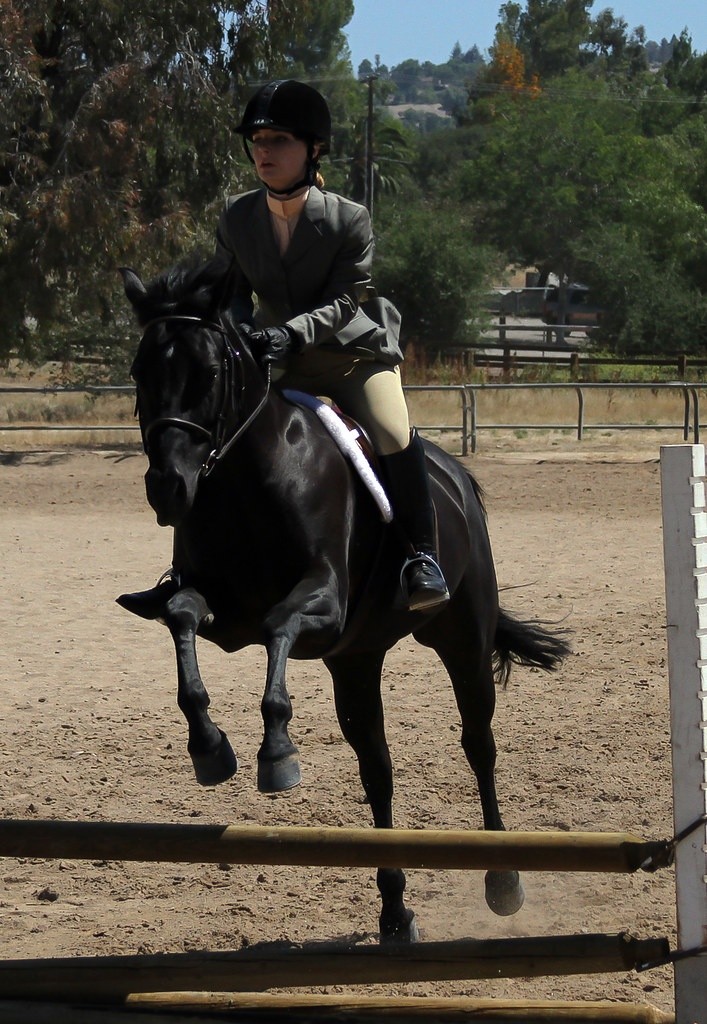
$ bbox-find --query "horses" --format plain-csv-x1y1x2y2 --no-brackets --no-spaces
109,259,578,948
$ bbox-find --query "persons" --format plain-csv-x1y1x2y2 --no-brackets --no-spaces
214,81,450,612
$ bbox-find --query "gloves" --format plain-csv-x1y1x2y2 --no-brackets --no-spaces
249,326,295,363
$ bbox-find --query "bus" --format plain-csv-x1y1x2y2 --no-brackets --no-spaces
476,285,601,346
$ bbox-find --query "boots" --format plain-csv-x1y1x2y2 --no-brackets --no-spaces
377,427,446,602
117,581,176,623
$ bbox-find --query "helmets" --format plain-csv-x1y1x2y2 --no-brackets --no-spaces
229,80,333,142
